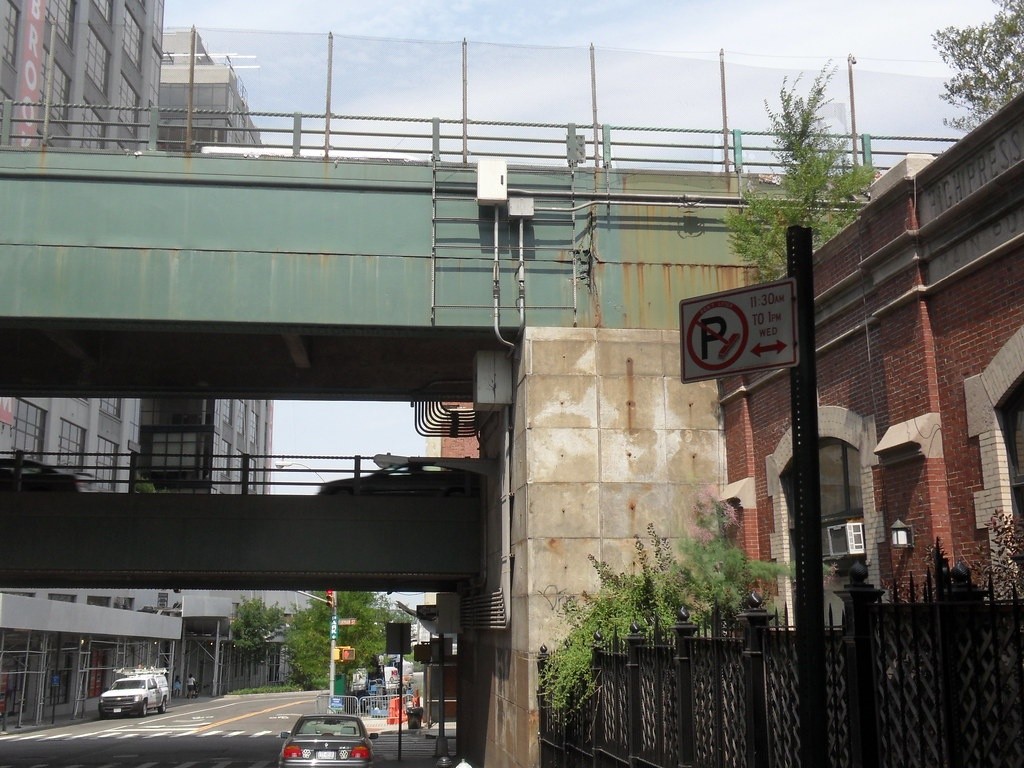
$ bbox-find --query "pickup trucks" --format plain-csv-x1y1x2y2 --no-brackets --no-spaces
98,674,169,717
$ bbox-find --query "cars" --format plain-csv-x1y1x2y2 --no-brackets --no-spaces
279,713,378,768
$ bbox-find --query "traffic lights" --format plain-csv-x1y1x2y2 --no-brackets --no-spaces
343,650,355,659
326,590,333,607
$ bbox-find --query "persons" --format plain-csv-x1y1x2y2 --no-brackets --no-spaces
186,673,197,700
174,675,181,698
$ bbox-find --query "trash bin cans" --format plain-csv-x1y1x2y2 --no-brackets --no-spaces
405,706,423,729
